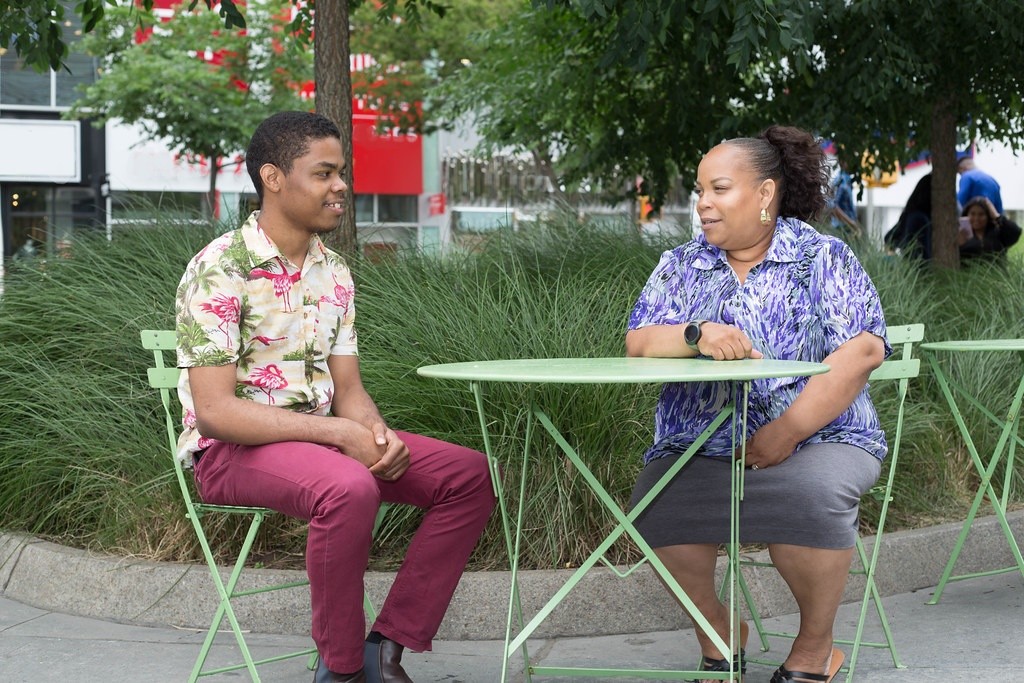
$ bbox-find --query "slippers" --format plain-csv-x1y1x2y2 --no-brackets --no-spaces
702,621,750,682
769,648,845,683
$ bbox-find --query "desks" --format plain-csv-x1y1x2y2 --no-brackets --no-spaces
416,358,830,682
920,337,1024,606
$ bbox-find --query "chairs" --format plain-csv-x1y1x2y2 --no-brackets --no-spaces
139,329,377,683
695,322,927,683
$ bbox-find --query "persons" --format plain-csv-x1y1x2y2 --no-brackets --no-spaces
829,152,1022,268
12,239,37,258
175,110,503,683
625,126,893,683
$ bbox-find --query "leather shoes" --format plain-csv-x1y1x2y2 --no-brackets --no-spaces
312,656,366,683
363,639,414,683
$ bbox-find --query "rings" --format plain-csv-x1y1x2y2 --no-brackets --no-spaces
752,464,759,471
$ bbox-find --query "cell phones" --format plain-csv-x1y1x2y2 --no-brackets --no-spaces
960,216,973,239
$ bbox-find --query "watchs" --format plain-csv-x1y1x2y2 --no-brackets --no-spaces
684,319,710,354
